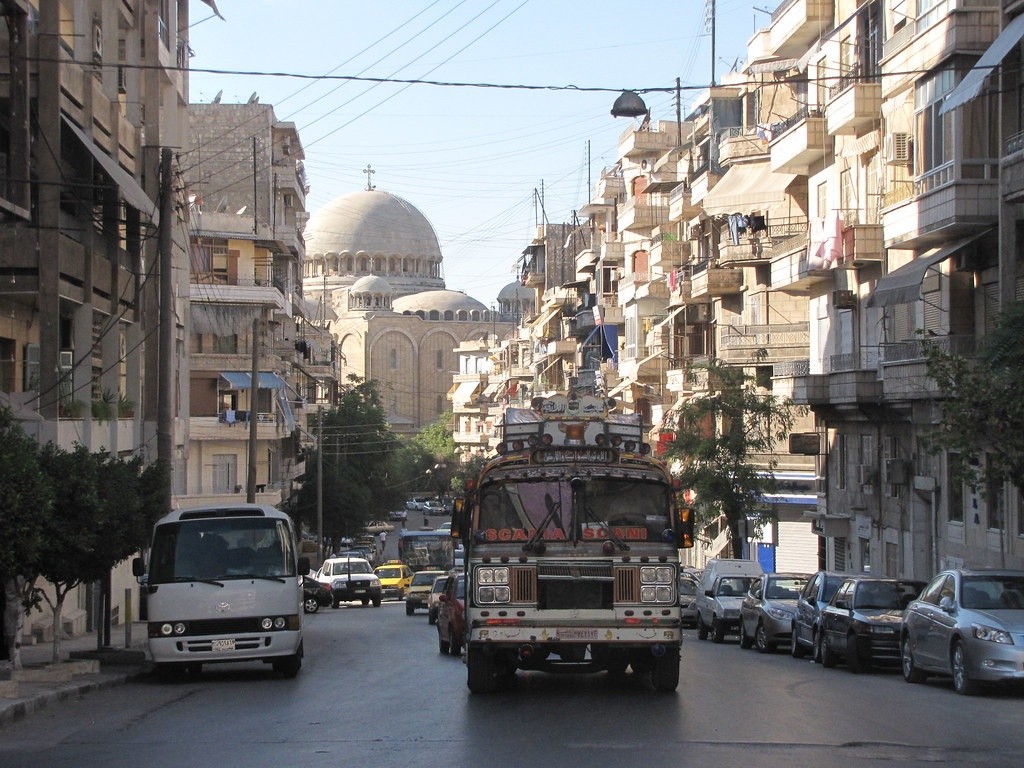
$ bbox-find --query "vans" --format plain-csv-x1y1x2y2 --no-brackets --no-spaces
695,558,763,644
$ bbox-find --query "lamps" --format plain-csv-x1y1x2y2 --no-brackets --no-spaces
610,91,650,119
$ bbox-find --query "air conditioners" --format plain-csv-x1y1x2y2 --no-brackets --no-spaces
92,24,102,58
855,464,871,485
883,132,909,165
117,67,127,94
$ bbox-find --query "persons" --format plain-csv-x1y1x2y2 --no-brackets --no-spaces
400,511,407,528
378,549,387,566
379,529,388,550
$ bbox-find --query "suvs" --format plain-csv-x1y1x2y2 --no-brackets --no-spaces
318,558,382,609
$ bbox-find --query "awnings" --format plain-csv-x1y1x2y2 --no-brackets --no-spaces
865,226,995,309
607,379,657,398
219,373,286,390
453,382,481,402
645,306,687,347
482,383,501,398
938,10,1023,122
629,349,668,396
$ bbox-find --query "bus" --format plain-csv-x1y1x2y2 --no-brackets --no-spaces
132,504,310,682
398,525,453,573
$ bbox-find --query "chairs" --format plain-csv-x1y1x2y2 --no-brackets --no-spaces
901,594,916,610
858,591,874,606
770,587,784,598
721,586,733,596
963,588,991,608
826,584,839,600
997,592,1021,609
168,543,279,577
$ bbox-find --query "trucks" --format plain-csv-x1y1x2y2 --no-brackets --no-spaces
450,393,696,697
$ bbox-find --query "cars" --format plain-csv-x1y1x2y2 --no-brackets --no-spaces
372,559,415,601
437,522,453,534
437,572,468,656
792,570,888,664
363,519,395,536
424,576,449,624
404,571,446,616
389,507,408,521
301,531,378,572
422,501,446,516
303,576,333,613
739,573,815,653
679,568,703,629
900,568,1024,697
406,496,427,511
454,544,464,572
818,577,929,674
